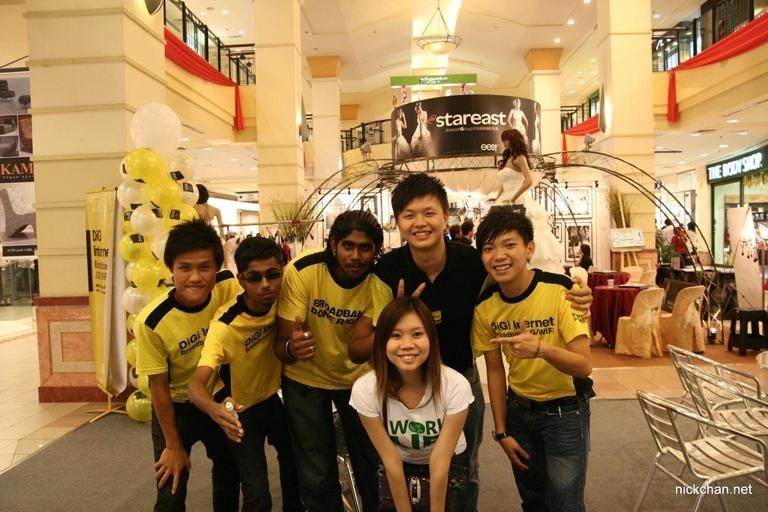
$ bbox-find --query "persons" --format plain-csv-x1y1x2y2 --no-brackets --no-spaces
194,184,223,232
394,109,412,159
140,221,240,512
577,244,594,271
411,102,430,155
655,216,698,267
375,175,593,512
460,221,474,244
348,297,476,512
472,209,592,510
488,129,567,276
449,225,460,240
274,210,427,511
508,98,528,144
188,238,297,511
532,102,543,154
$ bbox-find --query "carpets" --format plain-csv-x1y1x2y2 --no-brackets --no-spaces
0,397,768,511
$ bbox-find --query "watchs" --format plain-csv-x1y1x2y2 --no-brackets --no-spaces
491,431,508,441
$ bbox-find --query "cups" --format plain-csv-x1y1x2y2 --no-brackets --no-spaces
608,279,614,288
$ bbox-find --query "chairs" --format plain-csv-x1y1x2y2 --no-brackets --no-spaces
637,343,768,511
586,266,706,360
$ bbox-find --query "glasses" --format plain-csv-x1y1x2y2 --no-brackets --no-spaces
242,267,282,282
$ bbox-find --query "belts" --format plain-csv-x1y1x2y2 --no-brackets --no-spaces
514,395,576,410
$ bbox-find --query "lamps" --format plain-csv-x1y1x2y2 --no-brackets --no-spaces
415,1,463,55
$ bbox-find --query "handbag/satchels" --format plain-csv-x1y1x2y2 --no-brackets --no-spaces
376,465,465,512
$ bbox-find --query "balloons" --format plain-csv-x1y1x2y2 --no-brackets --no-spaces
114,103,200,423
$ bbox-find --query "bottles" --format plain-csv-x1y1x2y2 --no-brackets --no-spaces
225,402,238,423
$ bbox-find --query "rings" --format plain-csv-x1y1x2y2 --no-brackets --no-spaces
304,332,309,340
309,346,315,353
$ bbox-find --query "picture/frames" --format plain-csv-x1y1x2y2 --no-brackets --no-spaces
565,222,593,263
545,185,593,219
551,222,562,243
361,196,378,215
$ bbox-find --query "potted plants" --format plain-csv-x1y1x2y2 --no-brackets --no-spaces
655,217,685,269
251,182,324,260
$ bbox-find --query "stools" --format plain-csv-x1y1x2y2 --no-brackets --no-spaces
728,308,768,356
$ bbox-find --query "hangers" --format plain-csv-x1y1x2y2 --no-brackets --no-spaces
218,221,316,240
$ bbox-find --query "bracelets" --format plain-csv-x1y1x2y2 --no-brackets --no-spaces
285,339,295,362
535,340,540,358
